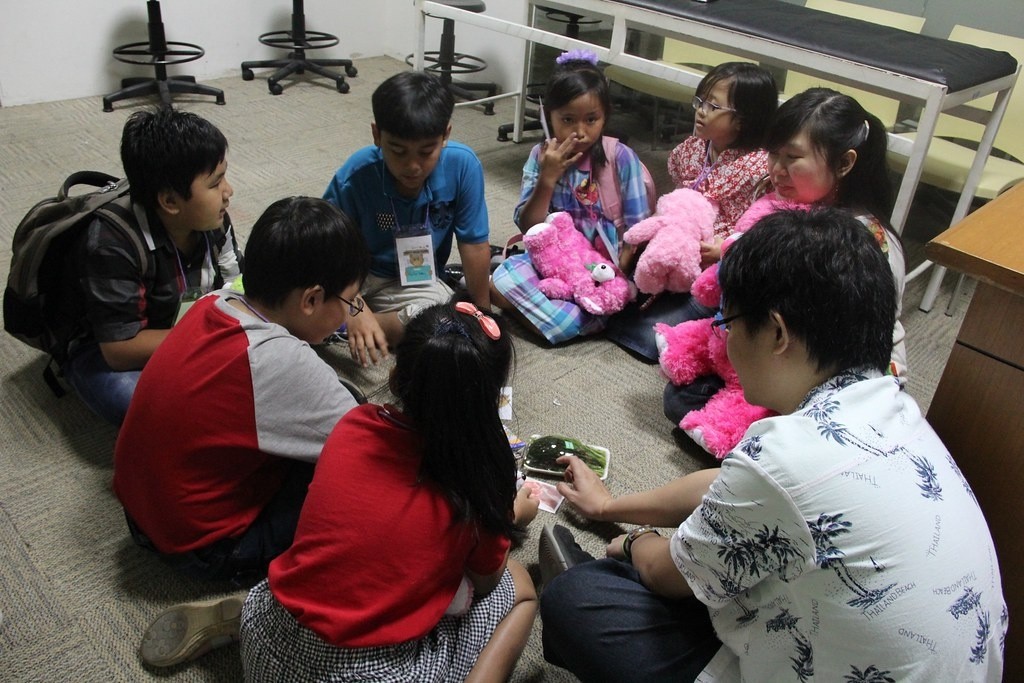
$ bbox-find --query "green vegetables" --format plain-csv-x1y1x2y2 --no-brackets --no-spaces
525,434,607,479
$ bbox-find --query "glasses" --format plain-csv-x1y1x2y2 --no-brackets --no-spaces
711,312,747,339
324,283,365,317
691,95,736,115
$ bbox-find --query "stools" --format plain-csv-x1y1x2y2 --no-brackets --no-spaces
494,5,628,144
402,0,500,116
239,0,359,95
102,0,226,115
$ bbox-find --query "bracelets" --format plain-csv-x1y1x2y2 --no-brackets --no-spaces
624,524,662,565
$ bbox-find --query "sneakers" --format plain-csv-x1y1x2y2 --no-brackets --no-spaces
538,523,596,587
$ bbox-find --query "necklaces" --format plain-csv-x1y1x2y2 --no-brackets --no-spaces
378,410,420,432
239,296,270,323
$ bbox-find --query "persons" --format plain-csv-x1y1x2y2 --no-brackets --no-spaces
664,86,905,461
110,195,372,589
538,206,1008,683
444,58,654,345
321,71,495,368
63,104,243,426
137,302,537,683
615,61,779,365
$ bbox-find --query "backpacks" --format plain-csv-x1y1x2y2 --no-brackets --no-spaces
3,171,227,356
502,135,656,259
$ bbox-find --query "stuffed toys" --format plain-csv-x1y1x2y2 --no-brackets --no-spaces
653,192,812,460
523,210,638,315
622,188,715,295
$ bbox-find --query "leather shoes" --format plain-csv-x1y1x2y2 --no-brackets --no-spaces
443,263,494,290
489,244,525,259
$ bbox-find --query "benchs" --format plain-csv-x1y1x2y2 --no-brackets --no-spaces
511,0,1022,316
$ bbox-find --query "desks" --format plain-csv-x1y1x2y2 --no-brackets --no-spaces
919,182,1024,683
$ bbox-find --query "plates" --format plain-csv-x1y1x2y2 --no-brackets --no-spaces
524,434,611,481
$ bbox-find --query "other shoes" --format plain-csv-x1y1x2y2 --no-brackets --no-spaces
138,599,244,667
338,376,368,406
323,323,349,346
